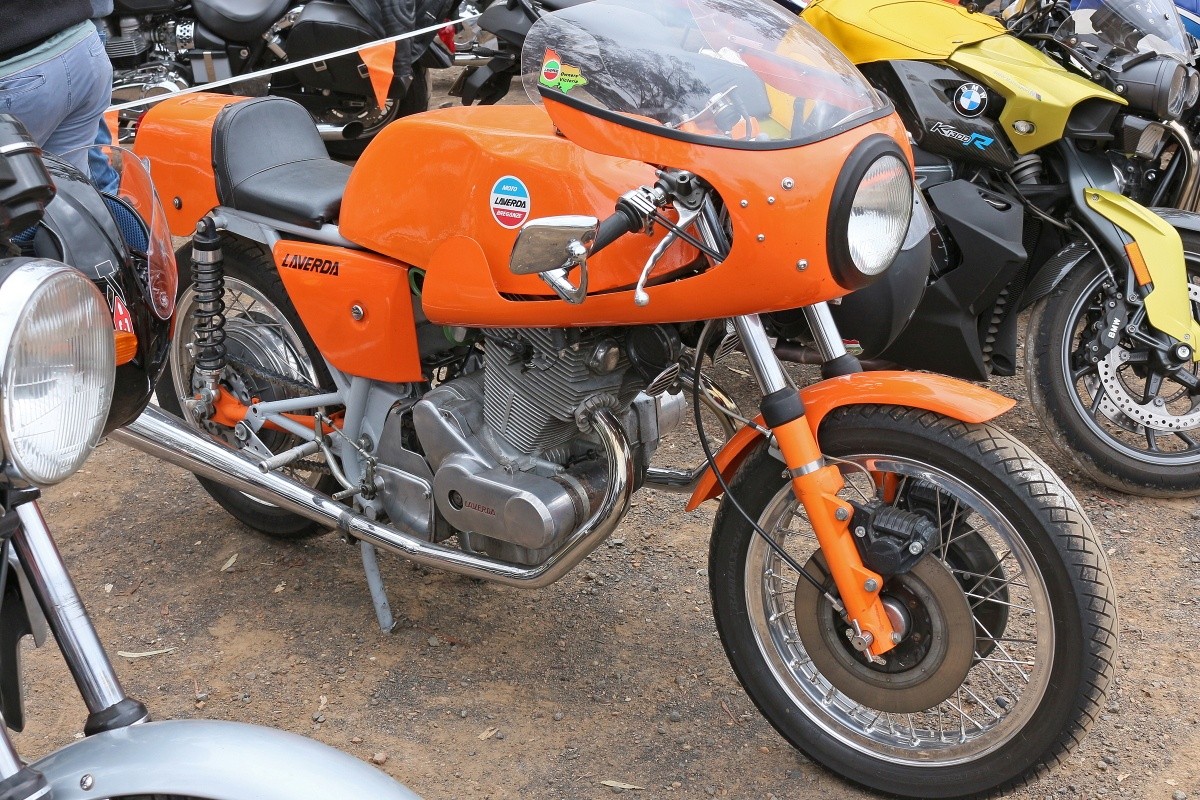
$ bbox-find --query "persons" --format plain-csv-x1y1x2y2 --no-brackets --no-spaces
0,0,113,178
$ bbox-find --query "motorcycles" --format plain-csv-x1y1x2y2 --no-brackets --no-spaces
93,0,1120,800
0,116,421,800
86,0,1199,500
